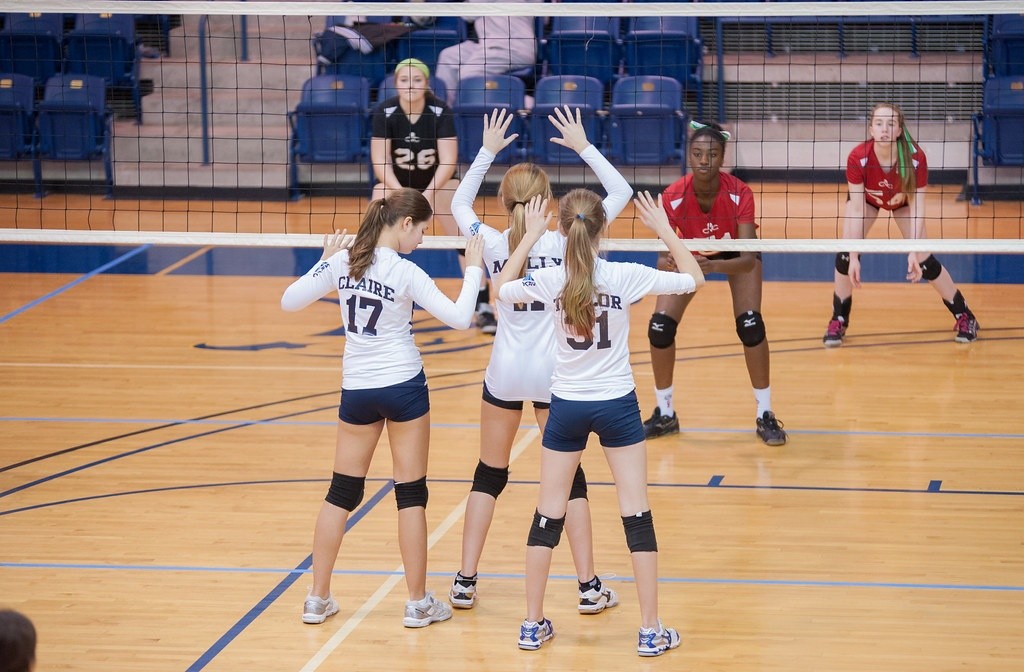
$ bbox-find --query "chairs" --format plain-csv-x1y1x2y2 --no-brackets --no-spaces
282,16,707,201
0,14,177,202
964,14,1024,207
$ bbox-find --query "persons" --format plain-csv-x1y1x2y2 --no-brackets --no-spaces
823,104,980,347
451,105,633,614
0,608,36,672
435,0,538,113
371,58,462,235
493,188,705,656
638,122,787,447
282,187,485,627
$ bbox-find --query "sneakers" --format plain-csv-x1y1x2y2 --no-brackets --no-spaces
301,594,337,623
578,582,617,614
450,576,477,609
474,301,498,334
953,313,980,342
637,624,680,656
517,616,554,650
643,407,679,438
823,313,847,346
403,593,452,628
756,411,786,445
